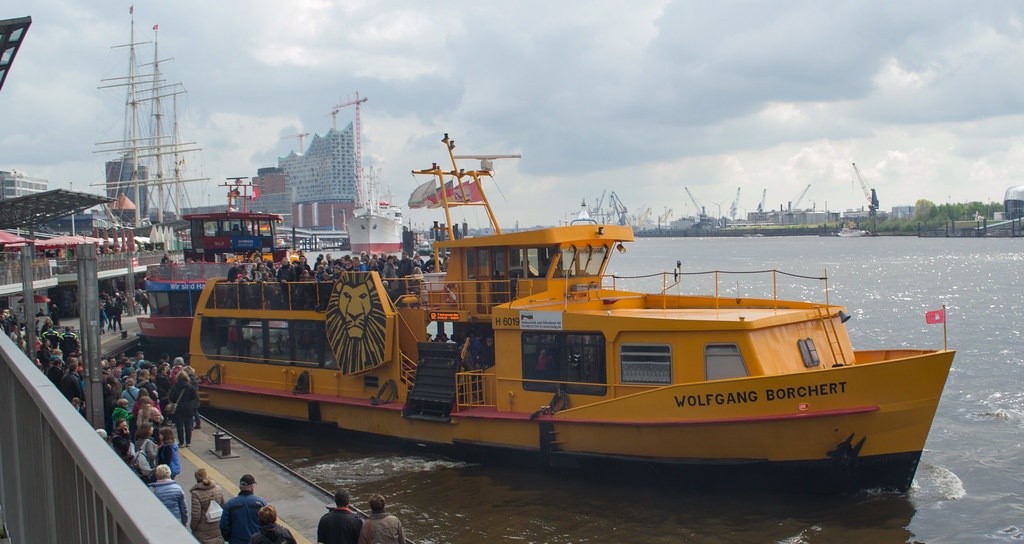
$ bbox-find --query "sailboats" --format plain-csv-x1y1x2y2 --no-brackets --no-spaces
89,2,212,249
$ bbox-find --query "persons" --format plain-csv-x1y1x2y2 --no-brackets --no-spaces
221,252,562,391
317,486,363,544
147,464,188,528
0,250,202,478
358,494,406,544
220,474,267,544
247,505,297,544
190,468,225,544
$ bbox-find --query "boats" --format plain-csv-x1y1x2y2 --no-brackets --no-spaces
569,198,606,254
137,173,304,354
346,165,404,254
416,242,434,256
837,223,867,237
188,131,955,494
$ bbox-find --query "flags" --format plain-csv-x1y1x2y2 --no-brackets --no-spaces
454,181,471,202
408,179,438,209
470,178,483,203
925,309,946,324
251,187,260,203
428,180,456,209
229,188,238,197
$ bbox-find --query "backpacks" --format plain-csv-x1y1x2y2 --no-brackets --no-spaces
136,439,157,478
202,488,224,523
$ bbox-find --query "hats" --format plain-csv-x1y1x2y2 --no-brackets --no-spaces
96,429,107,438
115,293,120,296
240,474,257,485
387,255,393,259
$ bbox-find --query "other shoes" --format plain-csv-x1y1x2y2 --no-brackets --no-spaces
178,444,191,448
194,425,201,429
100,328,124,335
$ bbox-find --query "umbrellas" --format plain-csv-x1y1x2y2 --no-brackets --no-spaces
0,225,174,255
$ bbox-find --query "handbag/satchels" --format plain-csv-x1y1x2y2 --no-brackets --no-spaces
164,403,177,415
127,443,135,455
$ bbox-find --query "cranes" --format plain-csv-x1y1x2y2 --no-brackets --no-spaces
281,133,309,155
674,193,688,219
325,109,339,130
685,188,710,231
758,189,767,222
852,163,879,232
789,185,811,212
588,190,673,229
332,90,368,196
730,187,741,220
